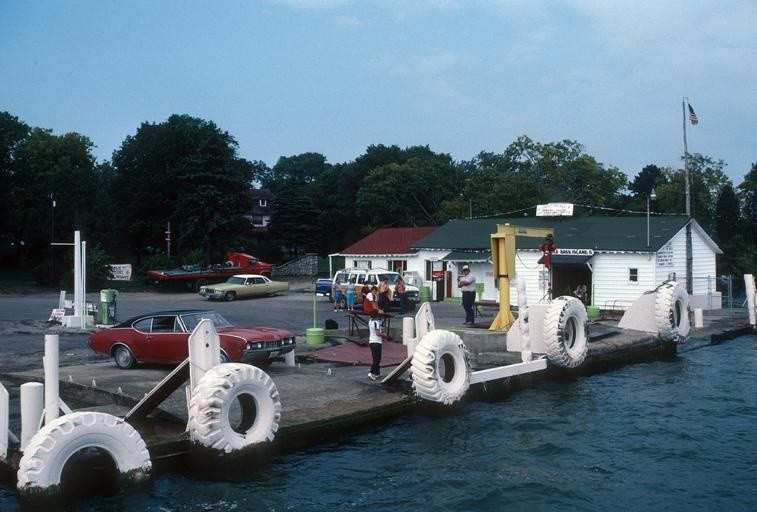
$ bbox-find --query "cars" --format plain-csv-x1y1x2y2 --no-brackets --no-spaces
86,307,296,369
197,273,290,303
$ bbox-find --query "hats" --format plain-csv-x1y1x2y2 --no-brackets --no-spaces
463,265,470,271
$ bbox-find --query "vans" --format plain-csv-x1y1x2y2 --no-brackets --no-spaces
331,266,421,310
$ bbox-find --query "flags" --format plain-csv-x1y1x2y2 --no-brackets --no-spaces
688,103,699,125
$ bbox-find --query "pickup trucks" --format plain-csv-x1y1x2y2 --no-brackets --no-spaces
314,277,333,302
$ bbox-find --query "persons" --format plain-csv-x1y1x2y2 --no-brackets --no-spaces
576,281,589,305
332,277,344,313
367,309,387,382
343,278,358,311
458,265,477,325
360,274,407,316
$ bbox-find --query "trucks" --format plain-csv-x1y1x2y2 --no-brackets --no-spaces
148,250,275,293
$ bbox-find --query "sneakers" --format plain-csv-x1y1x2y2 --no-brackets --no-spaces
348,308,354,312
462,321,475,325
368,372,380,381
334,309,343,313
